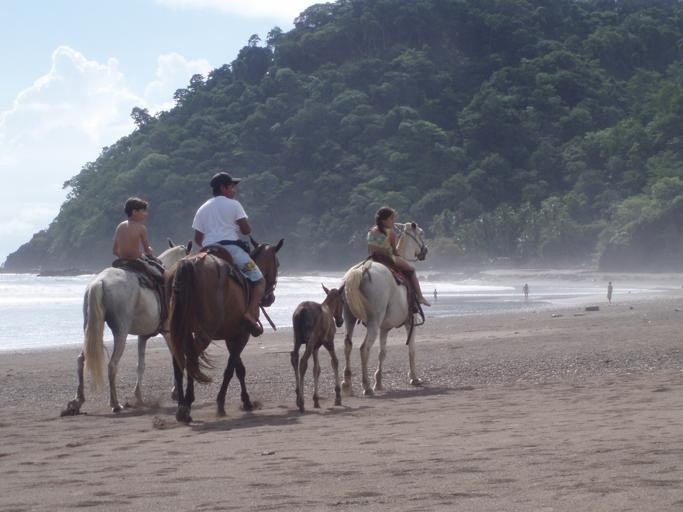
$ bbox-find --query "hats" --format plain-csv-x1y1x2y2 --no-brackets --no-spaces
210,171,241,186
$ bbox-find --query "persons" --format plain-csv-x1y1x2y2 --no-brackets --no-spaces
522,283,528,298
111,198,164,285
367,205,431,307
190,171,271,335
606,282,612,303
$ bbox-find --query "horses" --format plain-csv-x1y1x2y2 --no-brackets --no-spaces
291,283,346,412
339,222,428,397
165,235,283,423
67,238,192,412
